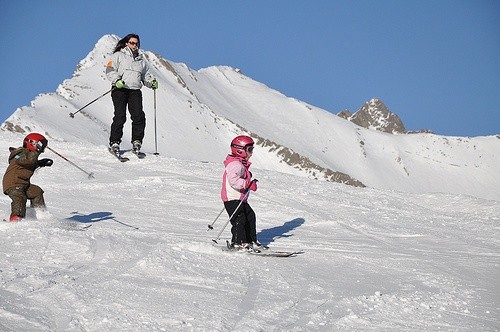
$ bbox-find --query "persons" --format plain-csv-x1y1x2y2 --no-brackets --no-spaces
0,131,55,225
220,135,263,255
104,32,159,152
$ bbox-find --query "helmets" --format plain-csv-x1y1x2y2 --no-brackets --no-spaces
231,135,254,158
23,133,48,153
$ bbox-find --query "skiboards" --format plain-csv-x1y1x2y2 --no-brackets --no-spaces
2,218,93,232
211,240,304,257
108,147,146,162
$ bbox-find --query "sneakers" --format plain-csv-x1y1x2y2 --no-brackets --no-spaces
253,240,269,250
230,242,261,254
108,143,120,155
133,140,141,149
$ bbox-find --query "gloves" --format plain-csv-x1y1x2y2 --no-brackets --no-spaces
35,139,47,152
247,181,257,192
151,80,158,89
38,158,53,167
115,79,125,89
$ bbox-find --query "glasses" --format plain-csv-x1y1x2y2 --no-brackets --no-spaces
231,145,254,152
128,41,140,47
